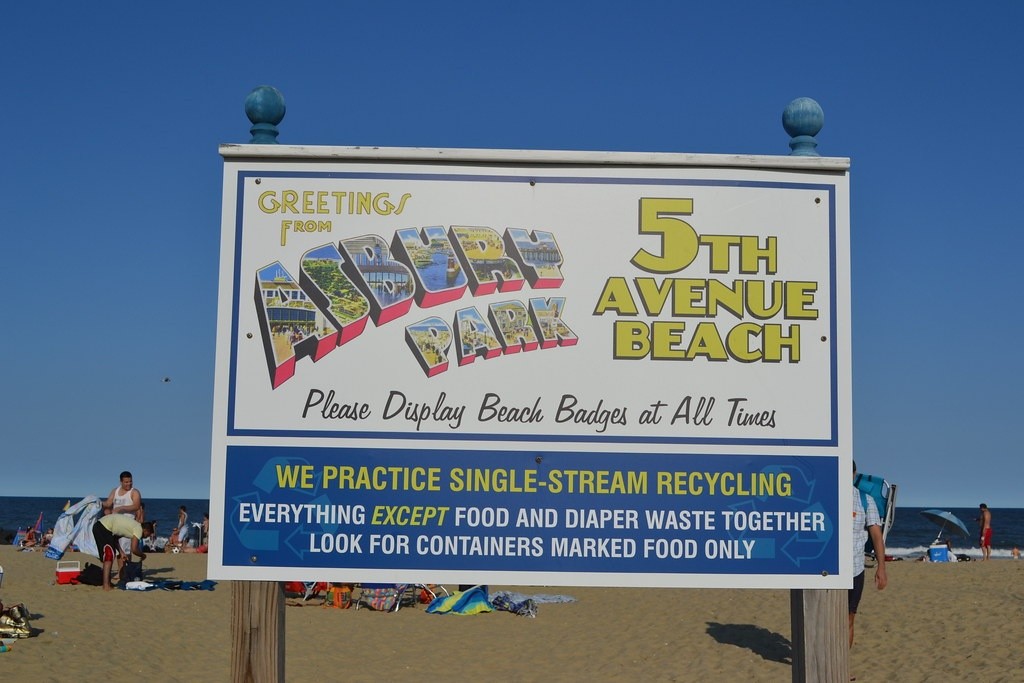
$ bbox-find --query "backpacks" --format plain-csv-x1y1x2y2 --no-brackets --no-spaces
323,585,353,609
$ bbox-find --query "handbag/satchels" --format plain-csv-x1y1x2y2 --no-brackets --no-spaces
119,558,144,582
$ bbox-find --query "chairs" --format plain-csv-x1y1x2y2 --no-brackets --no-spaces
280,579,451,613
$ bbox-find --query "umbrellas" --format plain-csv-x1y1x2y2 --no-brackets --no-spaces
919,509,971,544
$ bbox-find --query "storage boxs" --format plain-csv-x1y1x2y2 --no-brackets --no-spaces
929,544,949,564
55,560,81,584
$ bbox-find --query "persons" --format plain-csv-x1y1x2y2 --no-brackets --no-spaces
847,459,888,681
1010,545,1020,560
136,505,209,553
92,514,154,591
943,537,952,551
979,503,992,562
25,525,54,548
103,470,142,579
